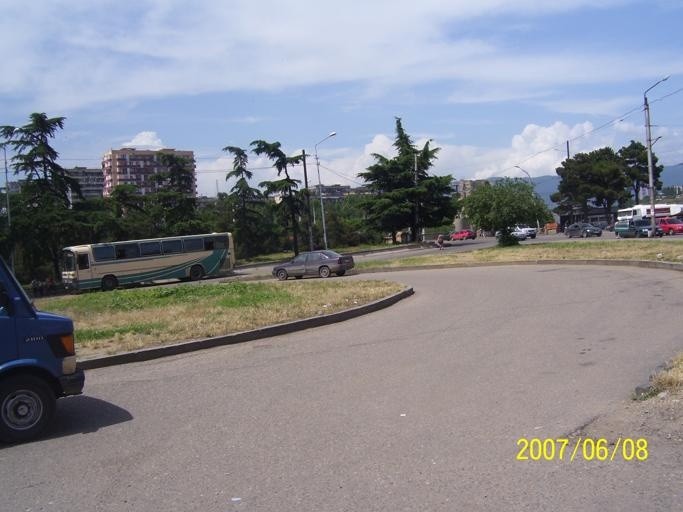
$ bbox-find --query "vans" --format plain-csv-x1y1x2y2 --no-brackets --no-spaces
587,214,615,230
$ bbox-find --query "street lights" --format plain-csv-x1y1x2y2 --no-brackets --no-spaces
414,151,423,244
314,132,336,250
513,165,540,233
642,75,671,236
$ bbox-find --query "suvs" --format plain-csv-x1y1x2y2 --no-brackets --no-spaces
613,219,662,237
0,254,84,446
513,224,536,239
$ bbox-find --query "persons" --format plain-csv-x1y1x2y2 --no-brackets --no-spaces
477,226,488,240
614,219,620,239
392,232,397,244
431,233,445,250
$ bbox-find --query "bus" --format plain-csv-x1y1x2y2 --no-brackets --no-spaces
60,231,236,292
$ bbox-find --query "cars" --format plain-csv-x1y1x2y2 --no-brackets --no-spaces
432,231,451,241
449,229,475,240
656,217,683,235
564,222,602,239
494,227,526,240
271,249,355,280
606,222,615,232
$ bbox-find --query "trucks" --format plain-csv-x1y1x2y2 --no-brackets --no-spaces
617,203,682,222
